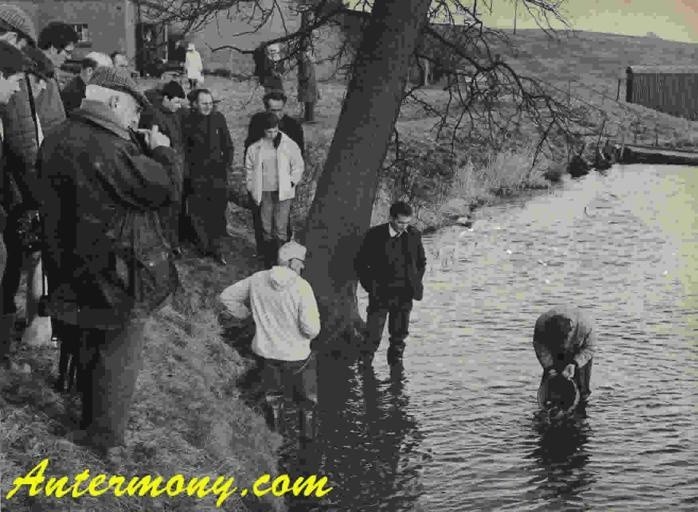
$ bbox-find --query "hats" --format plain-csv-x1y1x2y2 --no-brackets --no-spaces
87,63,154,111
21,44,57,86
0,3,40,47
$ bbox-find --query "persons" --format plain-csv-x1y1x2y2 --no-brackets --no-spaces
533,306,597,419
0,19,322,449
353,201,427,369
